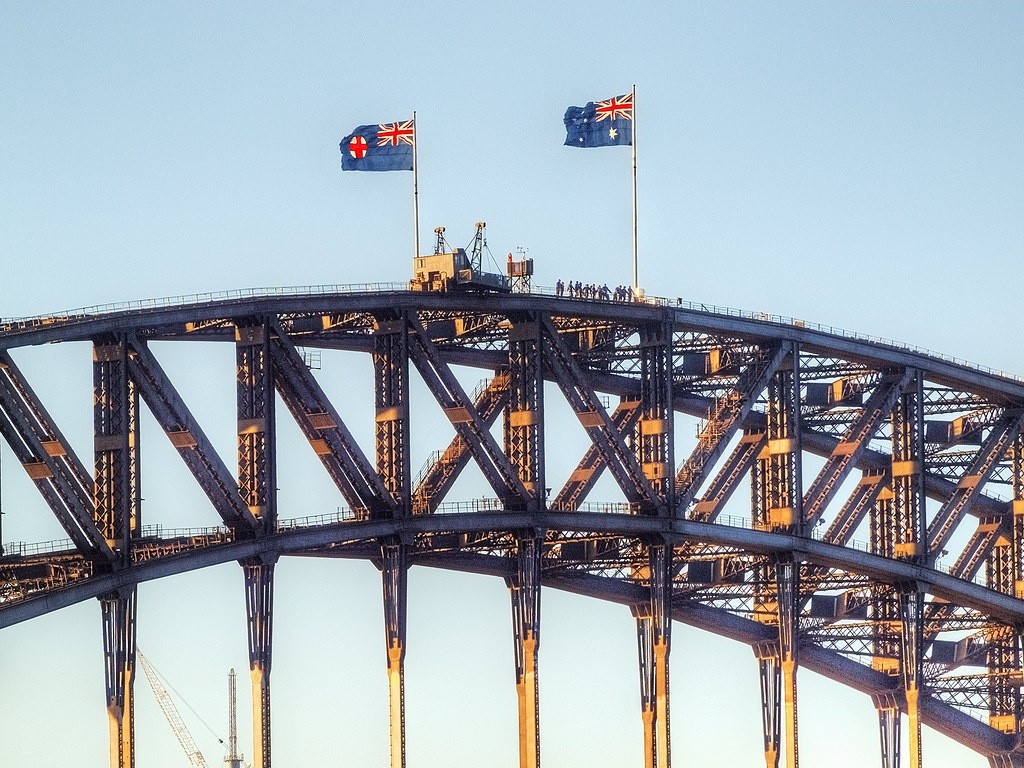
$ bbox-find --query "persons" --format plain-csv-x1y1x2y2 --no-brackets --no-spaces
556,279,635,305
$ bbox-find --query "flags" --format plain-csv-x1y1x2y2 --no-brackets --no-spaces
339,118,413,172
563,93,632,149
